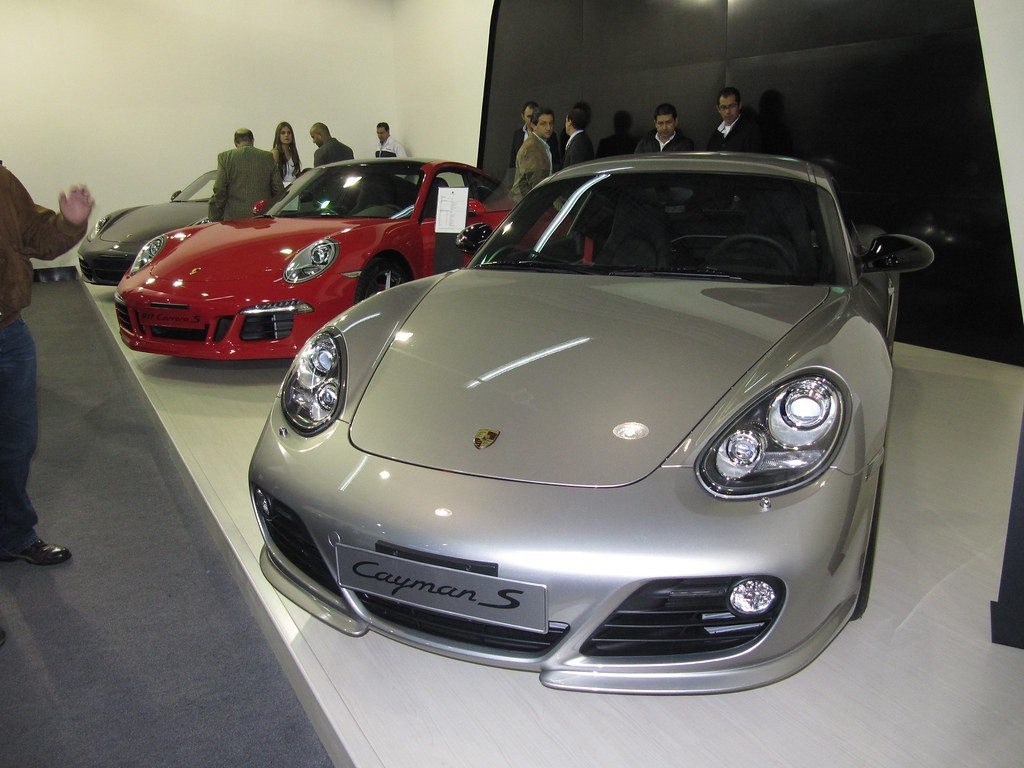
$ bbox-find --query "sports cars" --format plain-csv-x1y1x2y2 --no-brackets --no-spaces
78,169,300,285
114,156,594,360
248,150,935,697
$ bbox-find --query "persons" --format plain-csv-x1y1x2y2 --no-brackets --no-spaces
633,104,695,154
707,88,754,152
372,122,408,159
209,128,283,222
270,122,302,195
509,103,595,205
0,156,98,566
309,122,354,167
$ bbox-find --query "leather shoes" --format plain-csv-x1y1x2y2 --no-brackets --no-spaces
0,629,6,646
1,538,72,566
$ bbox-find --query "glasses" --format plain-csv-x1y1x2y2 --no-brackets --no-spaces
718,103,739,111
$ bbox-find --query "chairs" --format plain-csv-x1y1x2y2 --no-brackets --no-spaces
716,180,820,279
430,178,450,195
594,186,664,269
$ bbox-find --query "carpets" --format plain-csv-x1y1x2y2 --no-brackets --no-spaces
0,280,358,768
